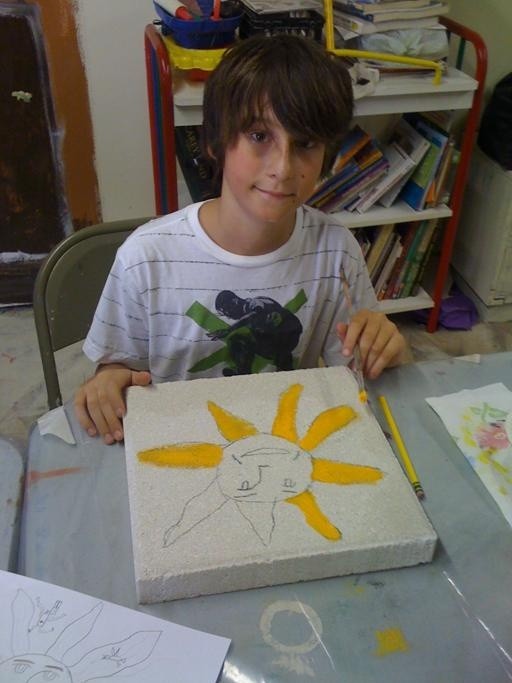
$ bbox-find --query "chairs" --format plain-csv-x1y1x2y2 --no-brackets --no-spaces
32,216,167,411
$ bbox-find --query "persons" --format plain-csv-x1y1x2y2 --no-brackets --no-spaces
71,31,413,447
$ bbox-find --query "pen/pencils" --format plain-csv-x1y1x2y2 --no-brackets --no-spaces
380,395,424,498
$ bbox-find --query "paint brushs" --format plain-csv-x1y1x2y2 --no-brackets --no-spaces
339,266,368,401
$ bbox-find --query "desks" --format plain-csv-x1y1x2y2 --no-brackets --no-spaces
24,351,510,683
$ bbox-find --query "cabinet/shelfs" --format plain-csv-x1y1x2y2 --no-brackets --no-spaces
142,18,491,332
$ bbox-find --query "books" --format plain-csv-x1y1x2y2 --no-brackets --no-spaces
175,129,222,202
325,1,452,77
305,108,458,300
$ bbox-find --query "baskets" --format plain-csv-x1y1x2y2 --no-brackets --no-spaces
239,6,325,49
